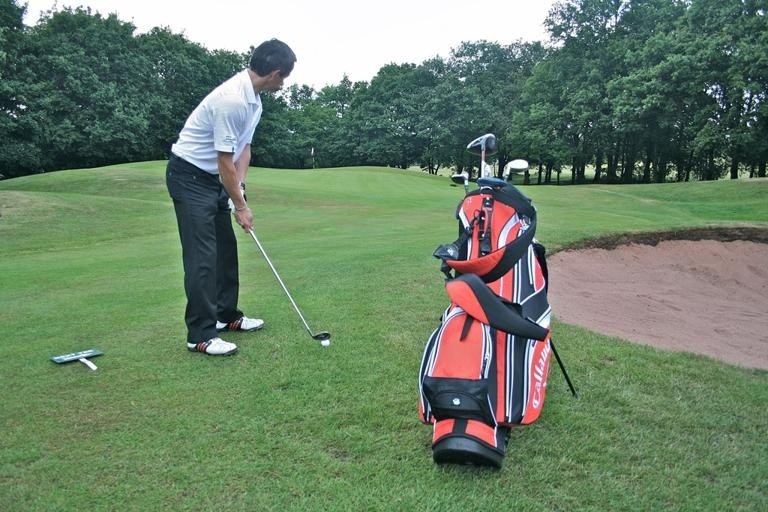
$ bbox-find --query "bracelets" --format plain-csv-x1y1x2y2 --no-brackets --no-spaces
232,203,248,213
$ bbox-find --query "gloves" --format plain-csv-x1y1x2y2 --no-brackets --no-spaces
227,186,248,214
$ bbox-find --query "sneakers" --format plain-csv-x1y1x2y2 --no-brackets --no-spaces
214,315,266,334
187,337,239,357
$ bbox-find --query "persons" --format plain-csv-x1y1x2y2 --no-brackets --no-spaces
162,37,296,358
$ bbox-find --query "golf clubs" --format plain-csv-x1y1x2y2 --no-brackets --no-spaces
452,134,528,193
246,228,332,340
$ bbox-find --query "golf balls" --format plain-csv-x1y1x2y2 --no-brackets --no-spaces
320,339,330,347
